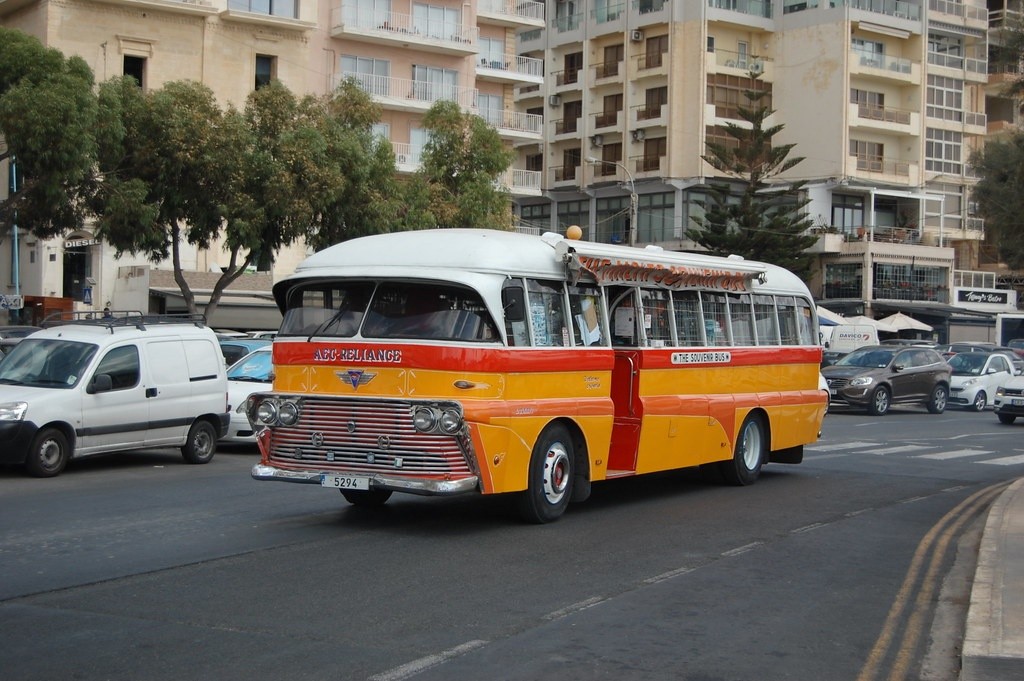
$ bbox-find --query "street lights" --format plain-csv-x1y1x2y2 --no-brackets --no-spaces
584,157,637,246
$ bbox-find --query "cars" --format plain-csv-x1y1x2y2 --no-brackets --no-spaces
1,324,43,360
209,327,282,441
882,337,1024,423
818,374,832,417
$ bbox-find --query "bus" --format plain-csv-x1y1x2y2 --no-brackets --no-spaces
242,226,832,527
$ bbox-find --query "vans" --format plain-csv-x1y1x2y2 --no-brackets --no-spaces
0,308,233,477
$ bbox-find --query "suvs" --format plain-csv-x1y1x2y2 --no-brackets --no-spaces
820,345,953,415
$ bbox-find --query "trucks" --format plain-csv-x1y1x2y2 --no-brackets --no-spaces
817,322,881,367
